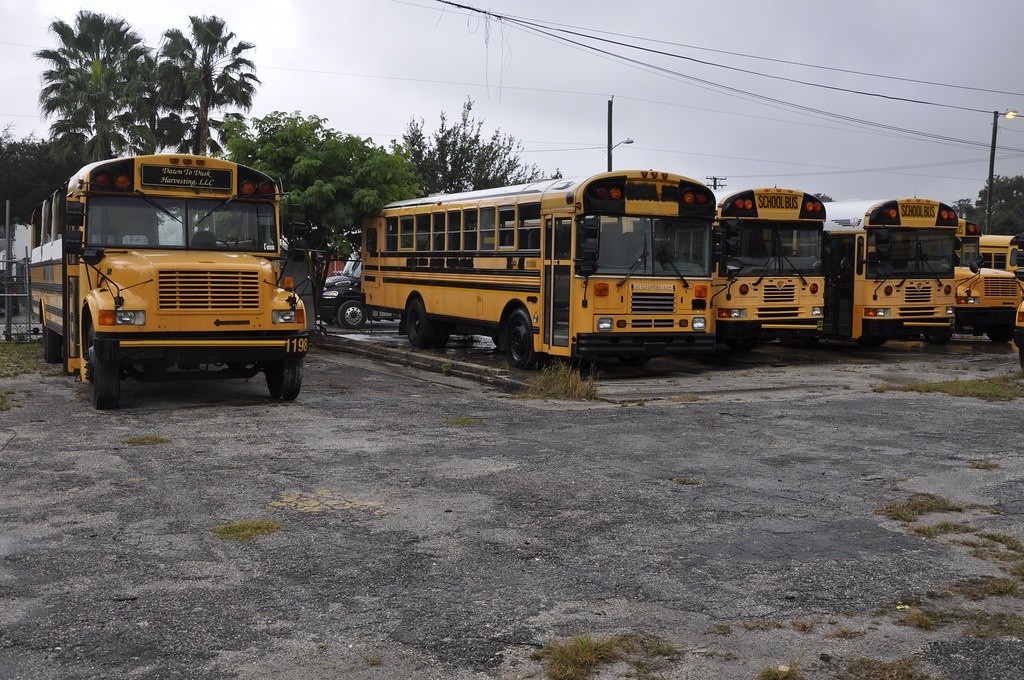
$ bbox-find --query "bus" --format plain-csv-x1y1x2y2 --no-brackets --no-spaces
361,170,1024,370
32,153,308,407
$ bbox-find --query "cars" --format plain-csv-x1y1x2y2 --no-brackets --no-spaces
316,251,403,328
0,271,21,315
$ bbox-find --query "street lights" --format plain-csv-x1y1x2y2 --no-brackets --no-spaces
607,94,633,173
986,111,1018,234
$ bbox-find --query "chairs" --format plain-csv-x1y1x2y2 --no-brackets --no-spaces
114,206,160,246
